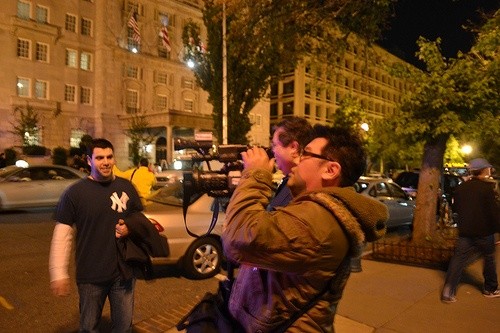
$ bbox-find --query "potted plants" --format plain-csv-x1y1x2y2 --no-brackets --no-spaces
22,145,50,166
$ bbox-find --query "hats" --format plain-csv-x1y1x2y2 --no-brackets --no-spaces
469,158,490,171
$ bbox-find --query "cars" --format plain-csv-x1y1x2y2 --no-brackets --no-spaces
151,143,251,198
139,184,237,279
0,164,90,214
352,176,416,235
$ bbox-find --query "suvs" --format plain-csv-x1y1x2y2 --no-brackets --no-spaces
393,168,470,220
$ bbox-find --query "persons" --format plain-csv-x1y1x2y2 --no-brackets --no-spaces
381,158,500,218
48,138,144,333
221,116,389,333
440,158,500,303
19,153,168,218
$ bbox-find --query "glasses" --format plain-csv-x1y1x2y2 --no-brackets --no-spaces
299,148,332,162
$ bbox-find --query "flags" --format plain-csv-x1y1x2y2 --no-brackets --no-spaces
159,22,172,53
127,8,141,42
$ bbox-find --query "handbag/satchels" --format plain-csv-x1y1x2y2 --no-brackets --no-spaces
176,290,248,333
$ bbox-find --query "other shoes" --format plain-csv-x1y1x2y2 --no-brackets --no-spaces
441,294,456,301
483,290,500,297
